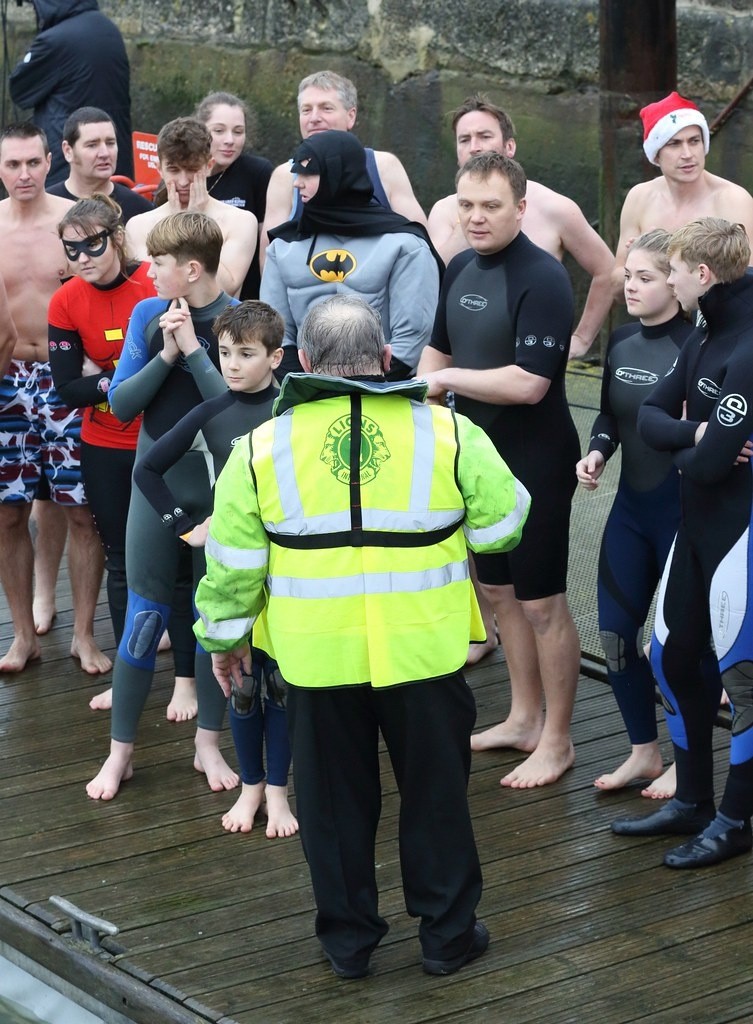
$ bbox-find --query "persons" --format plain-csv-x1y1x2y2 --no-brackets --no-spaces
0,1,616,976
575,92,753,871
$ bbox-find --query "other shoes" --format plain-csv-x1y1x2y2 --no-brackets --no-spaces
661,812,753,869
610,797,717,836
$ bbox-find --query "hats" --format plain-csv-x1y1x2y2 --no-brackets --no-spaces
638,91,710,167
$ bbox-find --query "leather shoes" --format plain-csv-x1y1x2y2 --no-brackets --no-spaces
420,919,489,976
321,949,368,977
